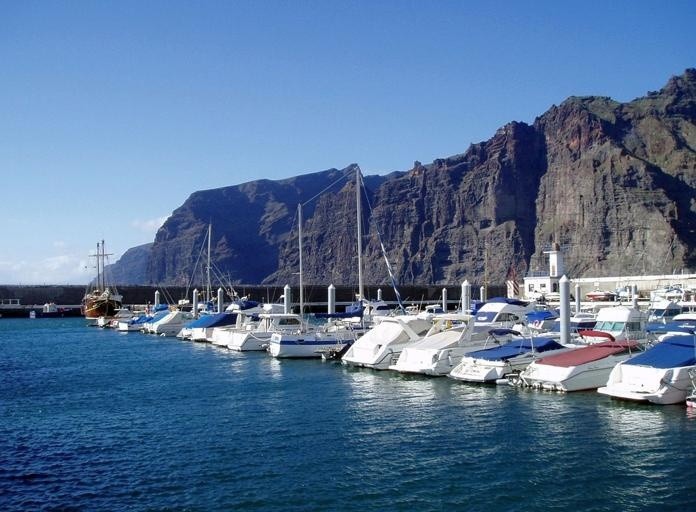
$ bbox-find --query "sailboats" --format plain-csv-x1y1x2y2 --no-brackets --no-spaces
82,240,123,319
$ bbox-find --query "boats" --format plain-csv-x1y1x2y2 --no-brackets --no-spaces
0,298,81,318
